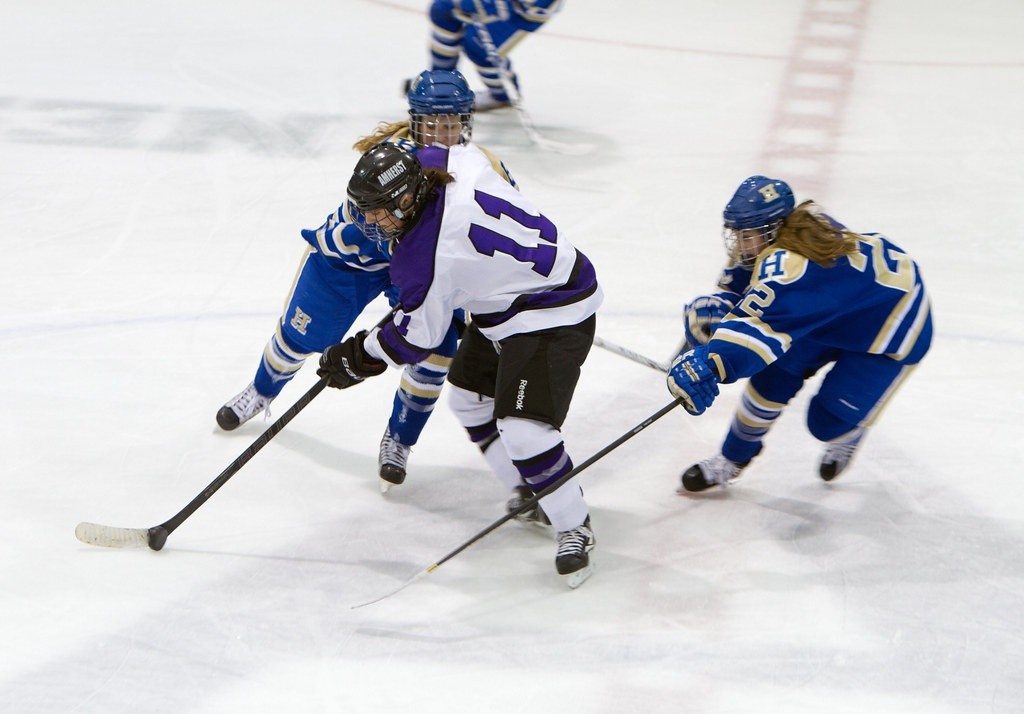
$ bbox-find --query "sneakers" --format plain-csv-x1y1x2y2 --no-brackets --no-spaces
506,483,552,529
475,89,517,112
556,517,595,588
820,433,860,480
212,383,278,431
379,424,411,493
677,441,762,494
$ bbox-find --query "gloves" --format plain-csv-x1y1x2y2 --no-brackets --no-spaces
667,345,719,415
317,331,388,389
683,296,735,347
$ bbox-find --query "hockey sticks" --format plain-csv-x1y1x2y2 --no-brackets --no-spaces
470,13,599,157
74,302,402,552
593,336,690,375
350,396,684,610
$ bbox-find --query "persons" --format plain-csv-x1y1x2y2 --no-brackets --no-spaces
403,0,566,112
319,139,605,589
666,175,934,496
214,73,476,492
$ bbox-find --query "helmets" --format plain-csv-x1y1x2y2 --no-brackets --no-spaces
408,70,476,149
723,175,795,272
347,141,429,243
